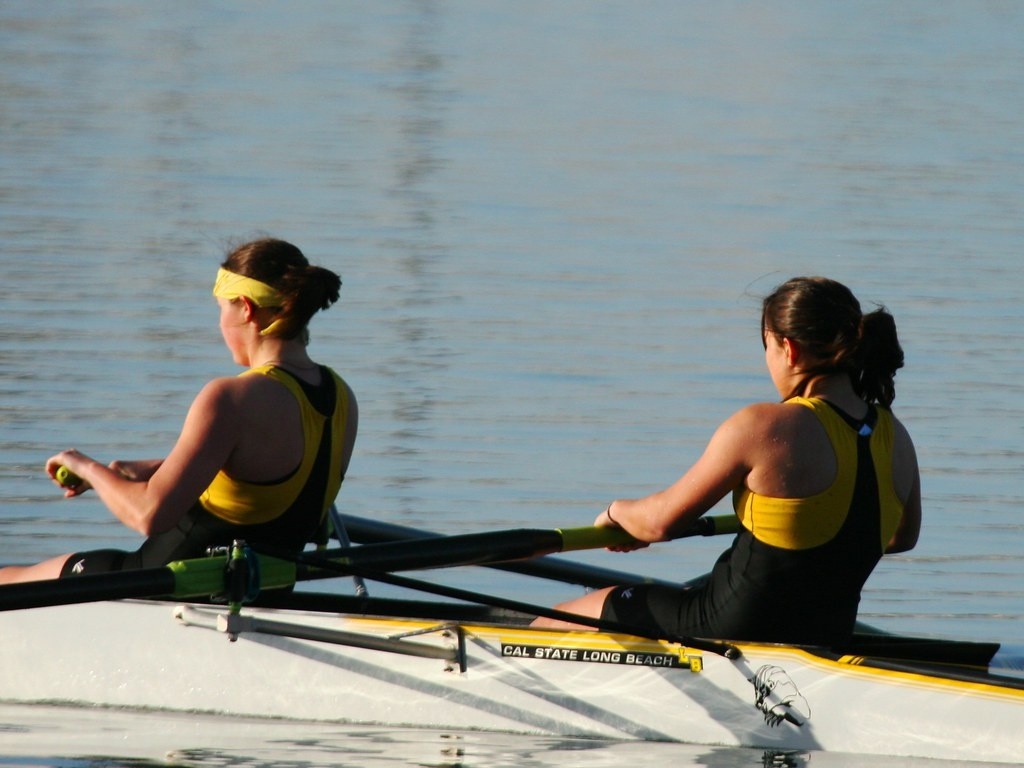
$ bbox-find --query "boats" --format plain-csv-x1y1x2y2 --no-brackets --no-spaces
0,575,1022,768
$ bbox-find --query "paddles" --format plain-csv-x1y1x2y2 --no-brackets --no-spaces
0,509,741,614
55,457,679,588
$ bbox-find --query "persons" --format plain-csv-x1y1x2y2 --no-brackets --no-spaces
529,276,921,653
0,240,358,596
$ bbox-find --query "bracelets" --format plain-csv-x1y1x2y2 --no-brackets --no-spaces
607,503,618,525
